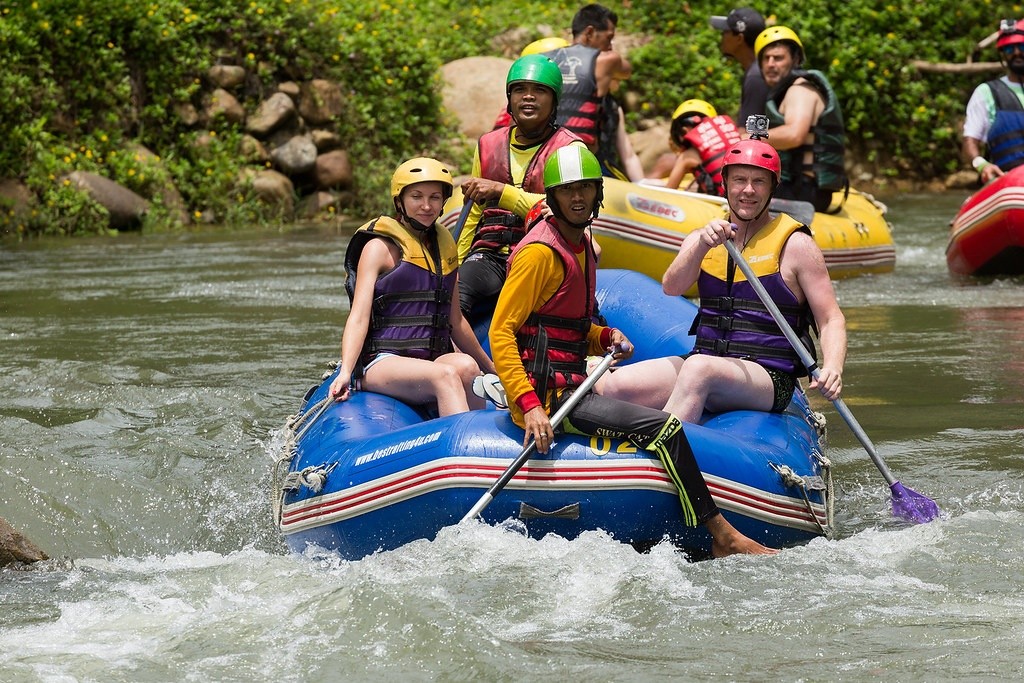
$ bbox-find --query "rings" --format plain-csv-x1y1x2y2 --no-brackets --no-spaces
710,232,716,236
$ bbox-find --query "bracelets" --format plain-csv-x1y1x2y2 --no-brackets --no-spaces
971,157,989,175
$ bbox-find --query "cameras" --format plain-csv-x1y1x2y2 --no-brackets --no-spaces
745,115,770,135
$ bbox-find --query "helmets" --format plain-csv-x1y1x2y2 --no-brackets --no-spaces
391,157,455,214
543,146,603,191
722,138,781,189
995,19,1024,48
669,100,717,155
507,55,563,108
754,26,806,66
520,36,571,59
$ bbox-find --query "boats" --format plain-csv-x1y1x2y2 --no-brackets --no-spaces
591,170,898,300
942,163,1023,277
277,270,831,557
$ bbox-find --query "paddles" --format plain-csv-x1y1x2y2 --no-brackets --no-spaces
453,187,486,245
718,222,940,520
458,341,631,524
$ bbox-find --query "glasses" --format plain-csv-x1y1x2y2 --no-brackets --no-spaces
1003,43,1024,54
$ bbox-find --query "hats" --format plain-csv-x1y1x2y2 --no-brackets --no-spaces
711,8,764,49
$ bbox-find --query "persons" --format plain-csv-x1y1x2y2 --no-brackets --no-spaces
329,158,498,419
435,4,850,364
488,146,783,560
963,20,1024,188
592,114,847,425
